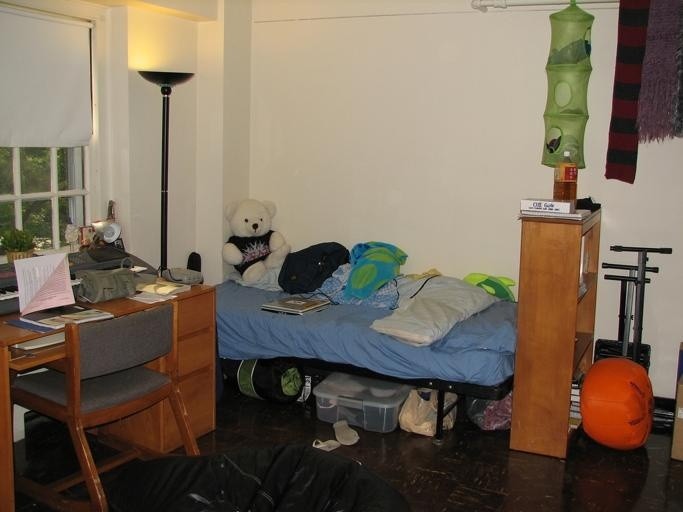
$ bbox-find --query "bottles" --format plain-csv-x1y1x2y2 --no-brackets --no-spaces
552,151,578,206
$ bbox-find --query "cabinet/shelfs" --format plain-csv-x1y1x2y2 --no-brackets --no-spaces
508,204,601,460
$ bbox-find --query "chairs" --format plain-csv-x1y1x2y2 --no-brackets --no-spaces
11,302,200,512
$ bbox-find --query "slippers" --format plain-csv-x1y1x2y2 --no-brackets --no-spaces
332,419,359,445
311,438,340,452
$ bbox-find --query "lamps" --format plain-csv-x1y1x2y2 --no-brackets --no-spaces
137,69,193,274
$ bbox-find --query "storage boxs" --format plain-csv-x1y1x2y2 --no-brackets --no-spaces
312,373,413,434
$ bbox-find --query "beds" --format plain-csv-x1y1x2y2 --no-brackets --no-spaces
213,277,518,447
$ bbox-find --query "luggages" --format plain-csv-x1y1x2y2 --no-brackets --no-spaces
295,363,329,411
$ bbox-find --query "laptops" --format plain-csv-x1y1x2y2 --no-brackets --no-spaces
261,298,329,315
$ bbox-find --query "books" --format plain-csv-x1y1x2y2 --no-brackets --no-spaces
568,370,588,431
124,280,193,305
258,297,331,317
2,303,115,334
518,209,593,221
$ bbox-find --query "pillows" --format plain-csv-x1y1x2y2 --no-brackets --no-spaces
369,273,495,344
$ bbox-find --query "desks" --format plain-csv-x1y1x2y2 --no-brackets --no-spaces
0,266,218,512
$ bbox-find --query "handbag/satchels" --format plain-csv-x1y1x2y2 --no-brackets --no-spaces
73,257,136,304
277,242,350,294
219,358,304,407
158,267,203,284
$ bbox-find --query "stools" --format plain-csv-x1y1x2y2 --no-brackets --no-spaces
580,358,655,449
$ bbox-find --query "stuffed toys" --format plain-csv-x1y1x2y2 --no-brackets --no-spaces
222,199,290,285
